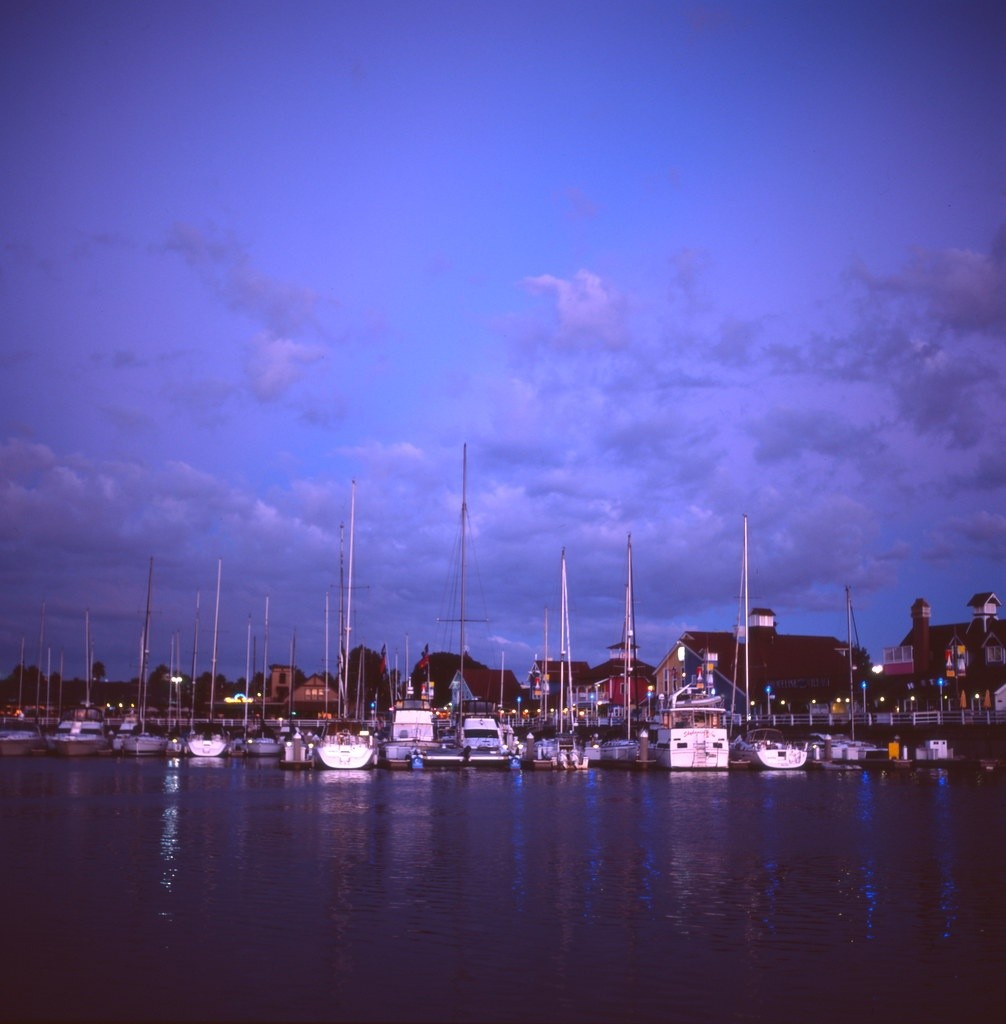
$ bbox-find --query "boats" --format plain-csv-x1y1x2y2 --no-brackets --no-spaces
911,739,1000,774
655,648,729,768
884,759,913,770
585,746,601,761
848,740,887,755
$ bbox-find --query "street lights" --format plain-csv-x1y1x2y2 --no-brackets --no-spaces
767,686,771,715
939,678,943,726
863,680,866,712
648,692,651,717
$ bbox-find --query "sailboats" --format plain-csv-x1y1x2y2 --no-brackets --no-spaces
0,637,50,756
276,478,379,770
804,583,867,759
599,533,638,761
381,442,522,770
534,546,589,771
166,557,226,757
230,595,283,755
45,607,108,755
729,512,807,769
112,556,170,755
